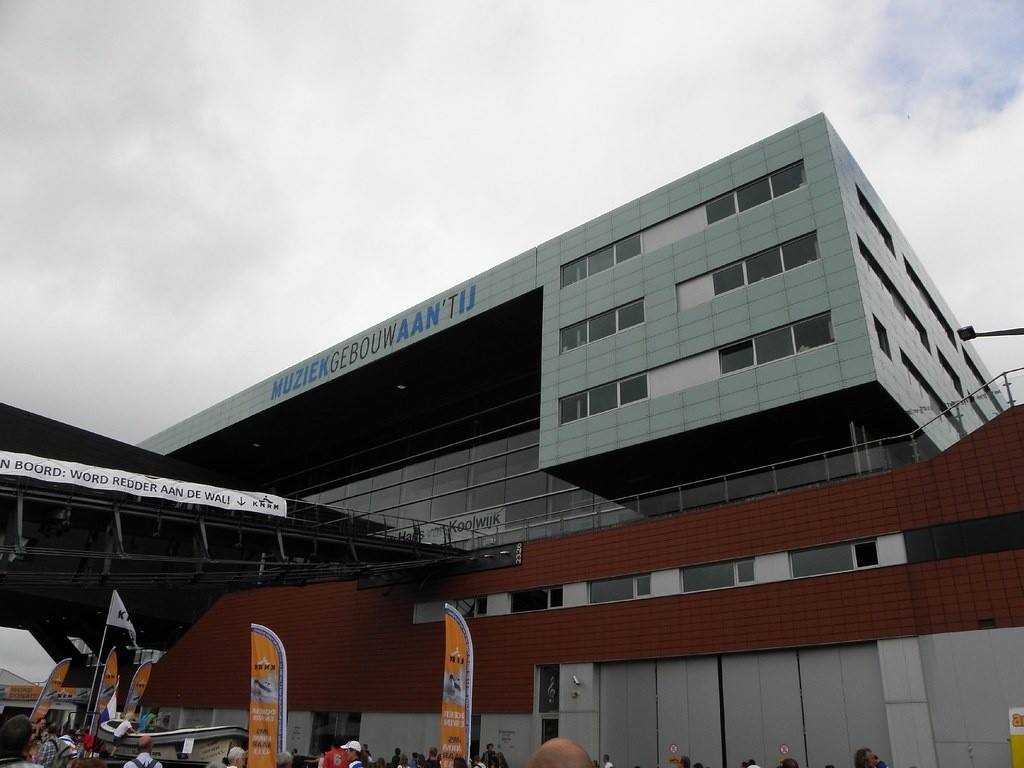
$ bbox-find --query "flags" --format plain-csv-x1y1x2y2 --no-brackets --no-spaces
108,590,140,650
99,683,119,724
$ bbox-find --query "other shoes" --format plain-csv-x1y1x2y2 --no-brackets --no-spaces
109,755,118,760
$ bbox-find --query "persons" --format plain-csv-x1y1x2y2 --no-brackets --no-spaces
529,738,592,768
0,705,890,768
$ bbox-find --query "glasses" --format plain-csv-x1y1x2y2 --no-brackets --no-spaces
344,750,352,752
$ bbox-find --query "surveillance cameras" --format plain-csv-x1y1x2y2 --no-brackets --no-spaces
573,676,580,685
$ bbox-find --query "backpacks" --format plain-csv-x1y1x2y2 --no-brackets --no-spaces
138,714,153,732
48,739,72,768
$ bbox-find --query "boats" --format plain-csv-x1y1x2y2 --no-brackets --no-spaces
96,719,249,765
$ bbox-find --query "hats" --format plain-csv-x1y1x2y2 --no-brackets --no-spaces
340,740,361,753
